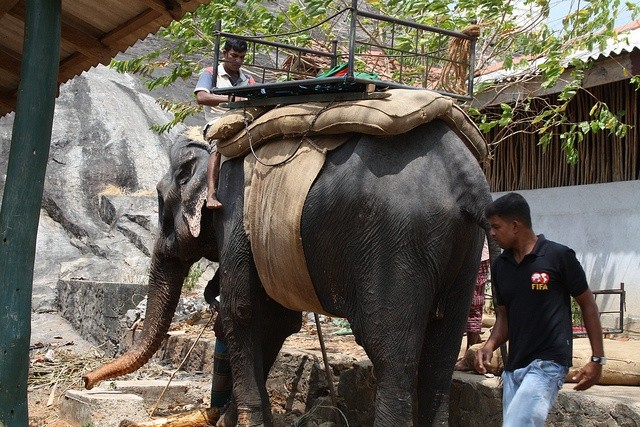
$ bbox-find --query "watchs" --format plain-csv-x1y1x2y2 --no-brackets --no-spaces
590,353,610,366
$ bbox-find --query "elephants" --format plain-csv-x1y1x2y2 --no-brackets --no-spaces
81,116,507,427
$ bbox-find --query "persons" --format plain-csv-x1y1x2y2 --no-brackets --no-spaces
454,225,492,373
473,191,605,427
193,35,260,211
